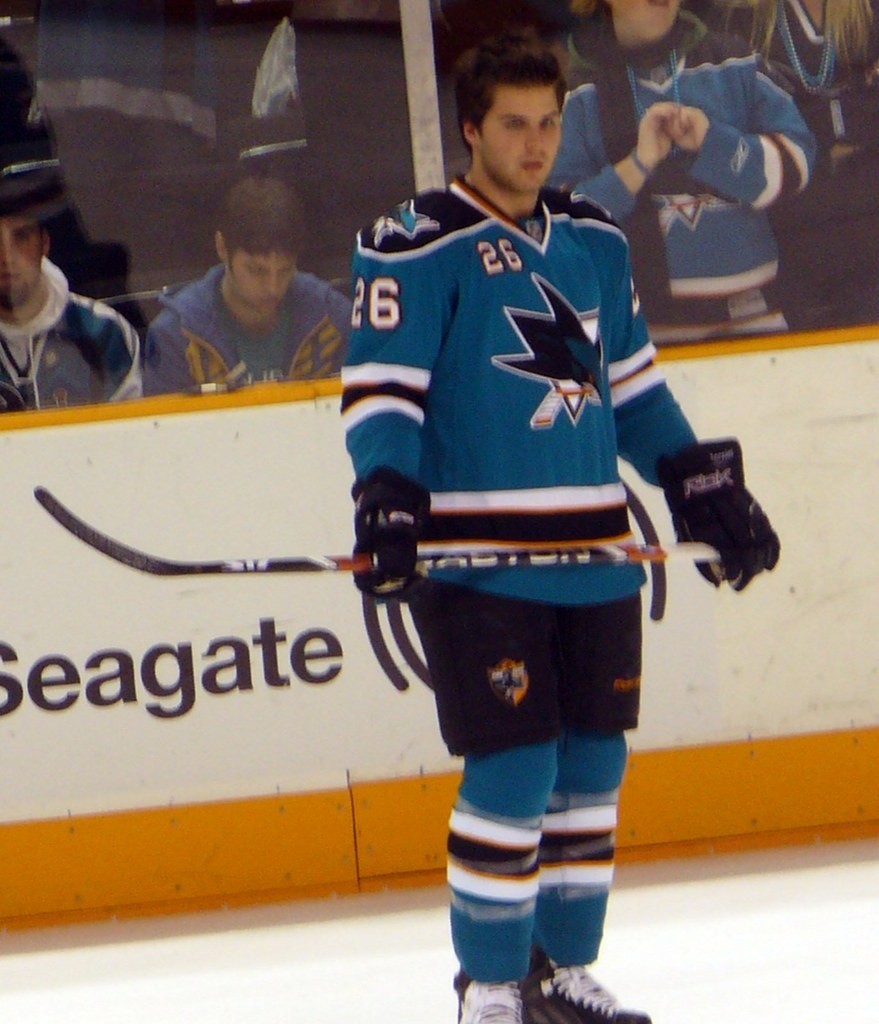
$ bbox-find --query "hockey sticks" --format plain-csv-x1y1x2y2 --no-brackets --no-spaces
34,484,724,579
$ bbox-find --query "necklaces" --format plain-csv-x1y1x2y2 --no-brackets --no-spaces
627,49,680,119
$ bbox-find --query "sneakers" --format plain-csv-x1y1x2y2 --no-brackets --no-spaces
519,941,652,1024
454,964,526,1024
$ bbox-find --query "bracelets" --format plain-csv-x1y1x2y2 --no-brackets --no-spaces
632,147,651,176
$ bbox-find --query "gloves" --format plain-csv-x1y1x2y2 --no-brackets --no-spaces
658,438,781,592
351,465,433,602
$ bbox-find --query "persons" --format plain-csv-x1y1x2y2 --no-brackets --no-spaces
1,0,879,412
339,30,778,1024
